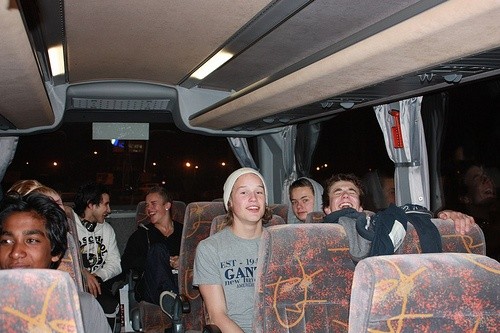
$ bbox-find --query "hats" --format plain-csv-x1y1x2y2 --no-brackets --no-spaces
223,167,268,211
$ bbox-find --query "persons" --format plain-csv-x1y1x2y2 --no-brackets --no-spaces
0,190,113,333
454,159,500,212
121,186,184,320
192,167,272,333
285,177,325,225
321,173,475,236
7,180,66,214
375,171,398,208
71,185,124,333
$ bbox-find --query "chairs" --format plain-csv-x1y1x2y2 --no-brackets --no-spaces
0,201,500,333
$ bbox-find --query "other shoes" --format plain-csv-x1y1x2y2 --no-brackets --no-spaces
160,290,177,319
